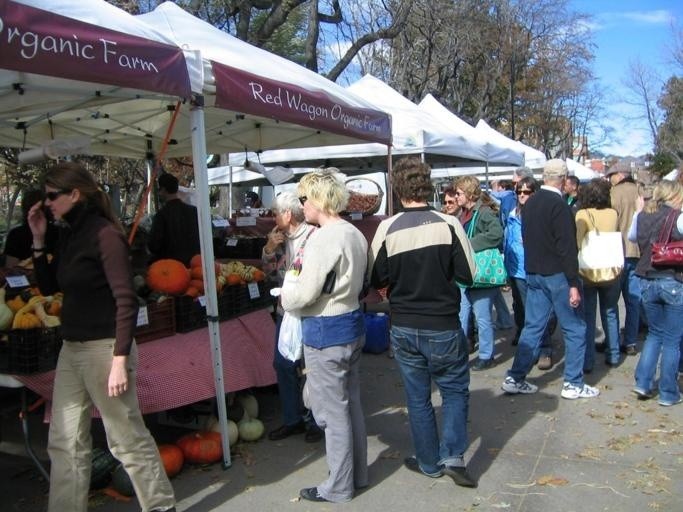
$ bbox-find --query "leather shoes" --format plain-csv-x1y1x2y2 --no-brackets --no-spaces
405,456,425,474
472,353,497,370
268,419,305,440
440,463,478,488
301,487,330,503
305,423,325,442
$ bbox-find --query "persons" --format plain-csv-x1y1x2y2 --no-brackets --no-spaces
567,180,624,373
145,174,204,257
440,188,471,227
5,191,67,261
365,158,475,486
280,171,373,504
502,159,599,400
559,176,577,208
28,165,179,511
261,193,323,442
501,176,556,371
489,180,519,216
606,162,647,355
628,181,683,406
456,175,508,371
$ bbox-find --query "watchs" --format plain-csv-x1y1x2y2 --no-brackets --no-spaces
31,247,46,254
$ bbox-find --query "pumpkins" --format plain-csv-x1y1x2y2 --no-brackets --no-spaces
179,432,223,464
228,273,240,285
157,444,182,476
240,267,254,282
214,421,238,445
215,262,219,272
236,266,247,273
217,283,222,291
246,265,256,271
191,267,202,280
239,417,263,440
186,287,199,297
228,260,243,272
148,260,189,295
255,271,264,280
217,275,226,285
242,396,259,417
191,279,204,294
221,268,229,277
220,264,228,271
191,255,202,267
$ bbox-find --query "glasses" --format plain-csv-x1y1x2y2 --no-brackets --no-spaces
443,200,454,205
43,191,67,201
271,212,277,217
456,192,464,196
299,196,308,205
515,190,533,195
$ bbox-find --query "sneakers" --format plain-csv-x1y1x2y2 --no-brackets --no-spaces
560,381,600,400
538,356,552,369
501,376,539,394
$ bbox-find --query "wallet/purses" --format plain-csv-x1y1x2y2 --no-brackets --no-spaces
323,271,336,294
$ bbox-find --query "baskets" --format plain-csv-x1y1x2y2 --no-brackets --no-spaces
343,178,383,217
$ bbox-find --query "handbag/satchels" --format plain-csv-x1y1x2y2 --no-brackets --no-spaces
456,205,509,288
575,209,624,271
651,208,683,266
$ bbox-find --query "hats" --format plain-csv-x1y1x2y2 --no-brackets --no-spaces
606,163,632,178
543,159,569,176
